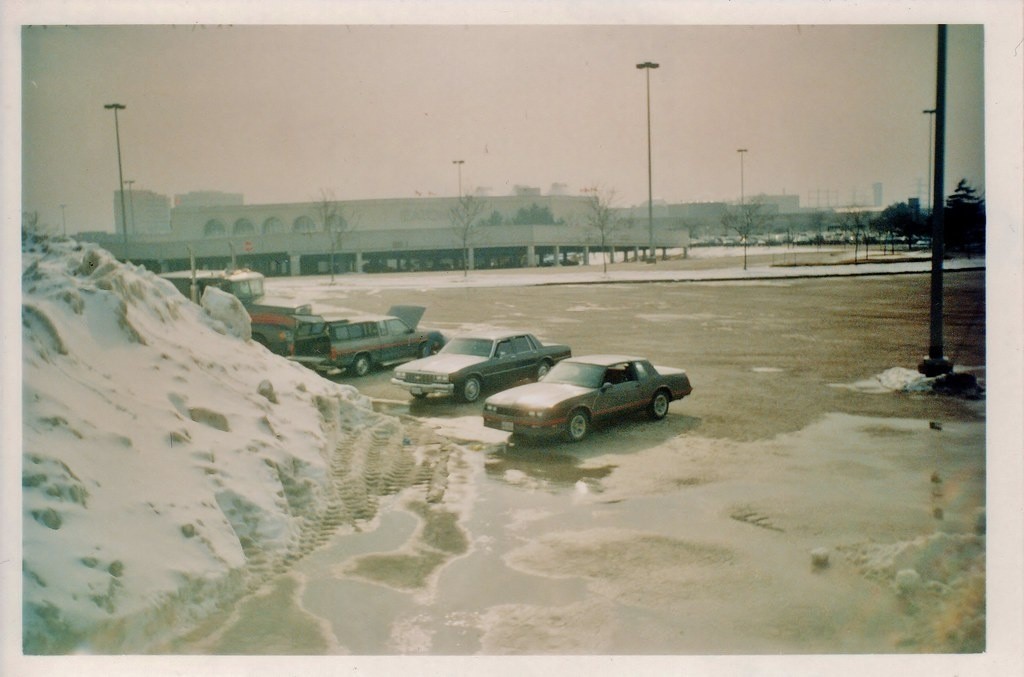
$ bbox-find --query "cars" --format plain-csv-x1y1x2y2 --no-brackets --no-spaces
482,354,695,443
389,328,572,405
690,229,929,248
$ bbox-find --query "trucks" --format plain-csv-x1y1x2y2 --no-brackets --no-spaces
157,270,313,359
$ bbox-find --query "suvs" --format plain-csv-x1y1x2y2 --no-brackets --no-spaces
285,303,446,379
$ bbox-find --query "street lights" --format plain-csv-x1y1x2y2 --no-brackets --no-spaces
637,61,661,261
452,160,465,202
923,108,936,223
104,104,130,260
737,148,748,271
